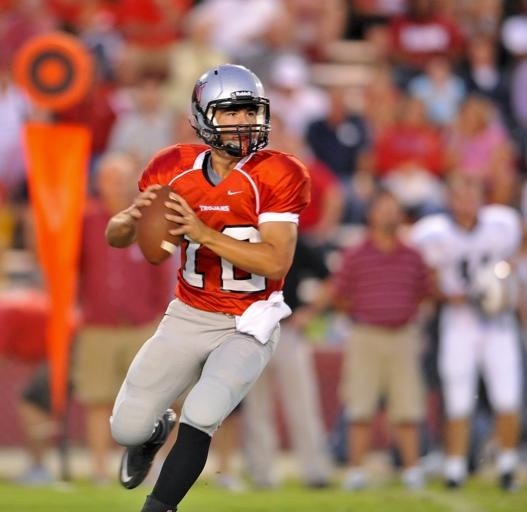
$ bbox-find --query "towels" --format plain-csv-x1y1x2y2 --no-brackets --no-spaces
235,289,292,344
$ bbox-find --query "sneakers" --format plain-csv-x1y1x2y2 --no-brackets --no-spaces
234,445,520,493
140,494,177,512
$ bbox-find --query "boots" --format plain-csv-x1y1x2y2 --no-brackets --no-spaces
117,408,177,490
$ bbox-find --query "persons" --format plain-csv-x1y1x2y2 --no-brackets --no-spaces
104,63,310,512
0,0,526,490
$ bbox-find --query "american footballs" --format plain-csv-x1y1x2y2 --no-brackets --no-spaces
137,186,184,265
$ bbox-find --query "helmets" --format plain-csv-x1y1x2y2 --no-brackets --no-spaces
188,64,270,142
